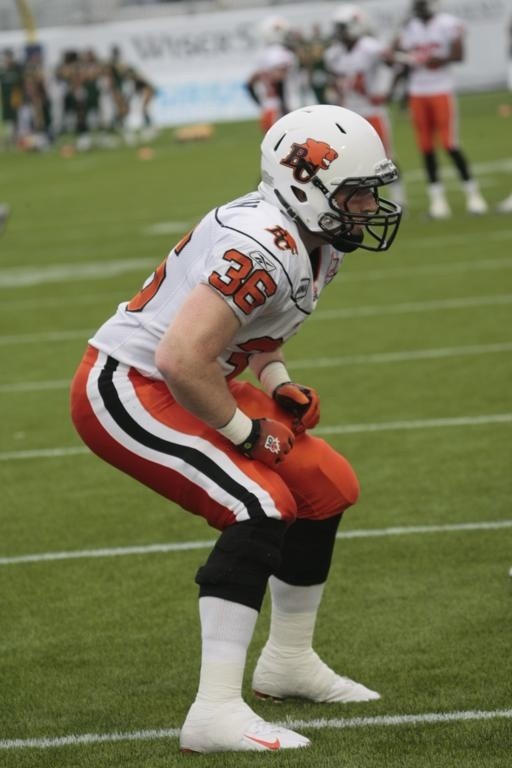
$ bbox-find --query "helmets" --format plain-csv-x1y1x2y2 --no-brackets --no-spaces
260,104,402,251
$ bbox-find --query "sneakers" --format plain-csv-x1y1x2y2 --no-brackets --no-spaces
429,195,450,219
252,651,382,704
179,705,311,754
466,198,487,213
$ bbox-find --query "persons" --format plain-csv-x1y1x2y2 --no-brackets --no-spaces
245,4,406,214
0,45,158,152
69,104,406,756
380,0,490,219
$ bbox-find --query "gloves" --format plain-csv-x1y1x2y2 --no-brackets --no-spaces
216,408,295,466
258,361,320,432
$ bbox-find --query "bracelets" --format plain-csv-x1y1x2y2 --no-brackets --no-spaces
215,410,255,448
258,361,290,398
